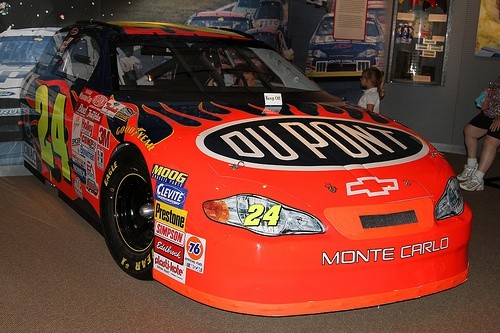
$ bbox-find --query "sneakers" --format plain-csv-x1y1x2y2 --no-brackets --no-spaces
457,164,478,181
459,176,485,191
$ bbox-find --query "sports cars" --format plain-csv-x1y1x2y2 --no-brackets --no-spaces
306,13,386,79
18,21,471,317
0,24,142,139
165,1,294,89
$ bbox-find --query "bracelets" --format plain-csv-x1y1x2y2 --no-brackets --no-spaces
497,118,500,126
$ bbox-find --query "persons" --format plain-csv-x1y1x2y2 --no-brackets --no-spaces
395,24,414,44
0,40,18,59
237,21,247,32
235,66,258,88
357,67,385,114
457,75,500,192
